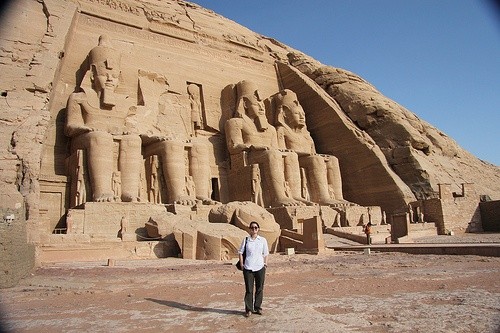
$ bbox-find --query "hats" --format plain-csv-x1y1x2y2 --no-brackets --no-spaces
368,223,372,225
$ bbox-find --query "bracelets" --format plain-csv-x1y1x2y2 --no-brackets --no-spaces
264,264,268,268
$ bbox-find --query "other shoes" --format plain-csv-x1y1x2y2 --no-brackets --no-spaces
245,311,252,317
256,309,263,315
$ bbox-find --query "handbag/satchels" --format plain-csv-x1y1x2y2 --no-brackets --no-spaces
236,236,248,270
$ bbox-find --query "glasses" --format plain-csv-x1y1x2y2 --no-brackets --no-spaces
250,226,259,229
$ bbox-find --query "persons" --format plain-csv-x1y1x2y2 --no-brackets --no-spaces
224,99,316,206
128,82,222,207
65,60,155,201
365,222,373,245
277,105,353,205
238,221,269,317
